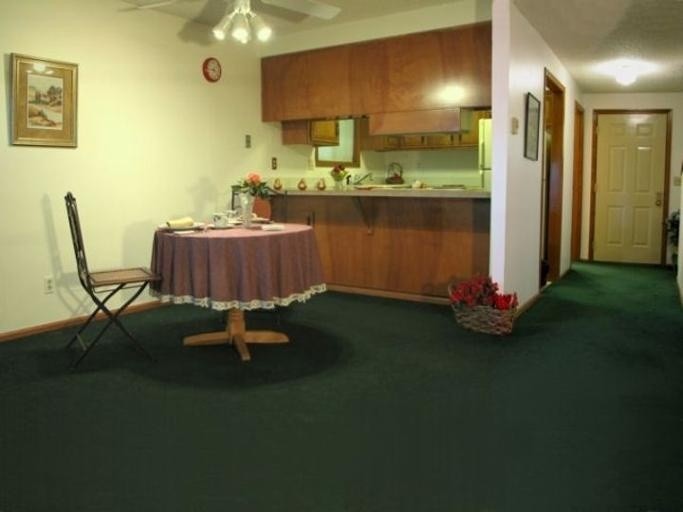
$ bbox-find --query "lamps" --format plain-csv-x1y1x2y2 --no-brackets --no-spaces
212,0,272,43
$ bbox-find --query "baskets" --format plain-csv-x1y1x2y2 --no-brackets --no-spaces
446,283,517,336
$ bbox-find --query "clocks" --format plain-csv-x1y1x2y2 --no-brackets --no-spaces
203,58,221,82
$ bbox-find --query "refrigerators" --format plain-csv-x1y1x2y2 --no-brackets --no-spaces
477,116,492,192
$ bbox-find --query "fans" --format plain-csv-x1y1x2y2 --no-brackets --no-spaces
137,0,341,21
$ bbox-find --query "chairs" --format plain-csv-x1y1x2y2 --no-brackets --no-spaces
64,192,162,368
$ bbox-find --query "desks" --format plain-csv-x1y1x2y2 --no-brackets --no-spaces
150,219,326,362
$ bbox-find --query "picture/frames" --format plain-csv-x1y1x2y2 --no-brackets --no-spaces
10,52,78,148
525,92,540,161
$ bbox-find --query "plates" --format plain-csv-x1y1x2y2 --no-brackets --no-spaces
155,192,283,235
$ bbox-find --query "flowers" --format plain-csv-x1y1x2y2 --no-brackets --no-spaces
449,273,519,311
240,171,268,200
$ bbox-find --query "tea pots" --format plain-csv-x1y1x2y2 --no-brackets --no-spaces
384,162,405,183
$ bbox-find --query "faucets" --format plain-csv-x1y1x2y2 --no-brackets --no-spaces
352,172,374,185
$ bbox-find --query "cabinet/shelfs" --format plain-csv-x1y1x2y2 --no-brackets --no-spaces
281,120,339,145
360,110,491,152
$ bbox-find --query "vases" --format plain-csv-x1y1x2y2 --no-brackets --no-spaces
238,192,255,227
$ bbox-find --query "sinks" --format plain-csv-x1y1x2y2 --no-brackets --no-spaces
353,183,410,189
